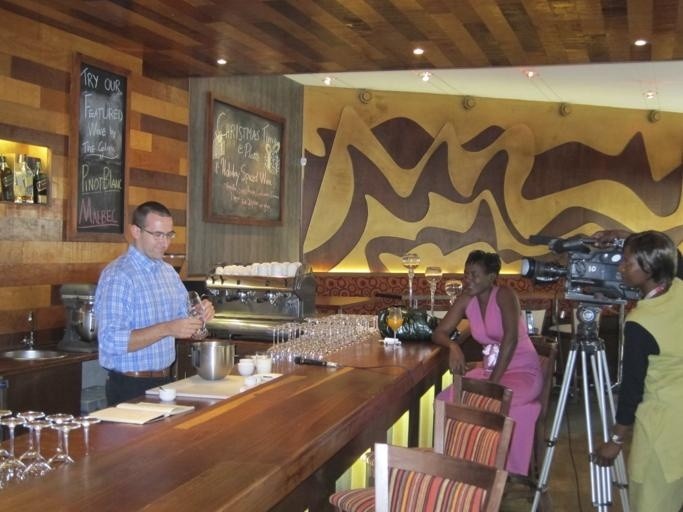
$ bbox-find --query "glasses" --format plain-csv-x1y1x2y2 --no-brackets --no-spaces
137,222,177,242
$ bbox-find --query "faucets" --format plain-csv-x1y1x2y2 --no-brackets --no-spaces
18,310,37,350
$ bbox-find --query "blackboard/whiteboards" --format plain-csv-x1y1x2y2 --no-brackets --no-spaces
201,90,294,227
61,48,134,243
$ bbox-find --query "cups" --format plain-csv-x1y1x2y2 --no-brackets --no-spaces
214,262,301,277
238,354,271,376
158,389,176,401
191,341,235,382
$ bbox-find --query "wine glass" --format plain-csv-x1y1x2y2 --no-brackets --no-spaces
387,307,402,345
0,406,102,486
423,266,443,318
445,279,462,306
402,253,420,309
267,312,378,368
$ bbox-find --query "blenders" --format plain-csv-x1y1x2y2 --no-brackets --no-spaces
54,284,98,354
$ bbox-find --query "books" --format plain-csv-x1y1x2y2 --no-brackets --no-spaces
88,400,194,426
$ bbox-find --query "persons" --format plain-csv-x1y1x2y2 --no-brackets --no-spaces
432,251,545,478
593,229,682,512
94,201,214,403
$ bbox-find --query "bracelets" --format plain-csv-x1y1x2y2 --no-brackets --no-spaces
447,341,455,347
611,434,625,445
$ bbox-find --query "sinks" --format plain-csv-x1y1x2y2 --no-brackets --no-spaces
0,348,69,361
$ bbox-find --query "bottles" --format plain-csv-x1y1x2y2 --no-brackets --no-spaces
0,152,47,203
526,309,535,335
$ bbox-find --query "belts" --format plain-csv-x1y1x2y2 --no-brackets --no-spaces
118,366,173,379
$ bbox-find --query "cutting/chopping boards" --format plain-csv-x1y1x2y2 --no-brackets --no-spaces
143,374,284,398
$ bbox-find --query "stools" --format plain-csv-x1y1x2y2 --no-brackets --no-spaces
374,442,508,512
507,339,557,512
452,373,512,416
328,400,515,512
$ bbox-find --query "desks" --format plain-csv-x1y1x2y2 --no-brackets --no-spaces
313,294,371,315
374,290,565,324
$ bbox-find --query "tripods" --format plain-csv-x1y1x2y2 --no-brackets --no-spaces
529,340,630,512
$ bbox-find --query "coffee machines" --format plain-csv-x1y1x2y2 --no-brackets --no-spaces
198,267,317,341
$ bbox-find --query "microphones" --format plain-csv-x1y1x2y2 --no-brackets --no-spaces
295,355,340,368
